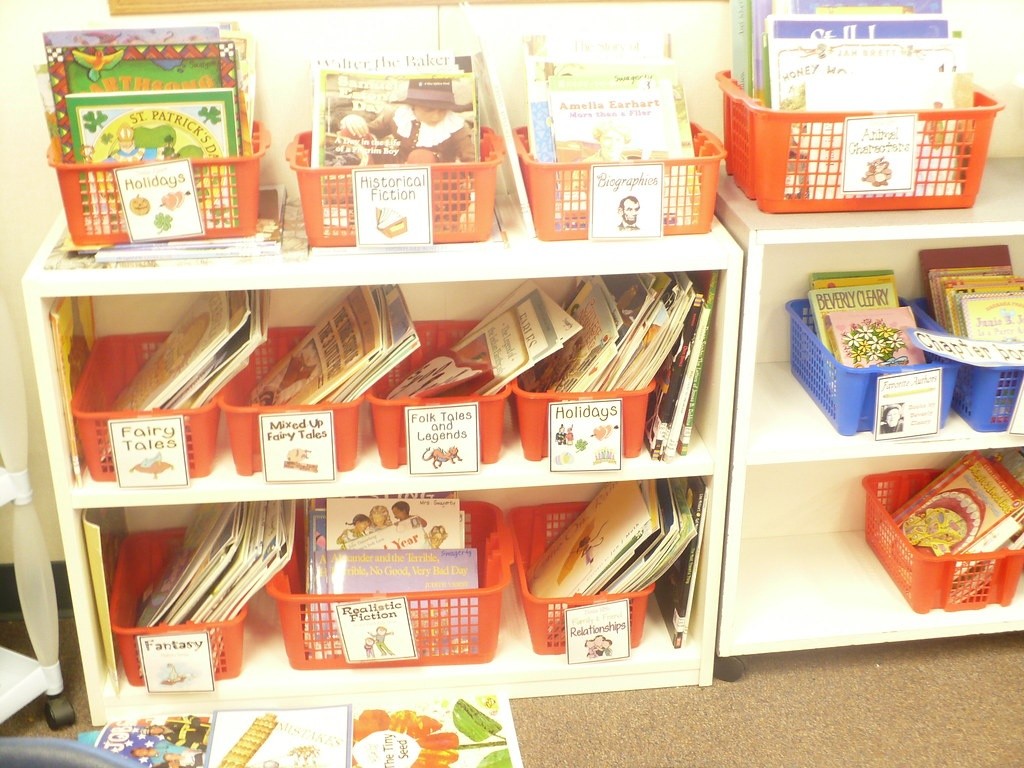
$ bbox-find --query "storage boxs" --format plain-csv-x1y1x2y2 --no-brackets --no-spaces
909,296,1023,432
512,377,657,462
108,527,250,686
514,119,727,243
47,121,271,246
363,320,512,469
264,501,512,671
285,123,507,248
715,67,1006,214
785,296,961,436
861,469,1024,615
217,326,363,478
505,502,655,655
71,331,220,482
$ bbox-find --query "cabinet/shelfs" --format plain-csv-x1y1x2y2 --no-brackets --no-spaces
0,297,76,732
714,156,1023,682
22,207,744,728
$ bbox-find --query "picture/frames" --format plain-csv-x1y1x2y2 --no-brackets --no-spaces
108,0,737,15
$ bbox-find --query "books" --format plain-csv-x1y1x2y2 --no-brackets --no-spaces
728,0,961,202
94,690,527,768
134,500,295,674
101,272,718,463
310,47,482,238
32,22,288,258
524,28,701,228
527,473,710,650
305,494,482,660
806,242,1024,422
877,446,1024,605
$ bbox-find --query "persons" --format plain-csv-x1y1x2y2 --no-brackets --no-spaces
340,80,474,224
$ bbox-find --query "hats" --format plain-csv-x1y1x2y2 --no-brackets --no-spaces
392,79,465,112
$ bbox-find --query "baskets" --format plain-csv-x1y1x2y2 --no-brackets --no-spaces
283,124,509,247
513,122,728,242
909,295,1024,434
716,68,1003,214
109,528,250,687
219,325,363,477
507,500,655,656
46,120,271,247
71,331,220,483
365,320,511,470
510,375,657,461
786,297,960,436
266,499,514,671
863,468,1024,613
